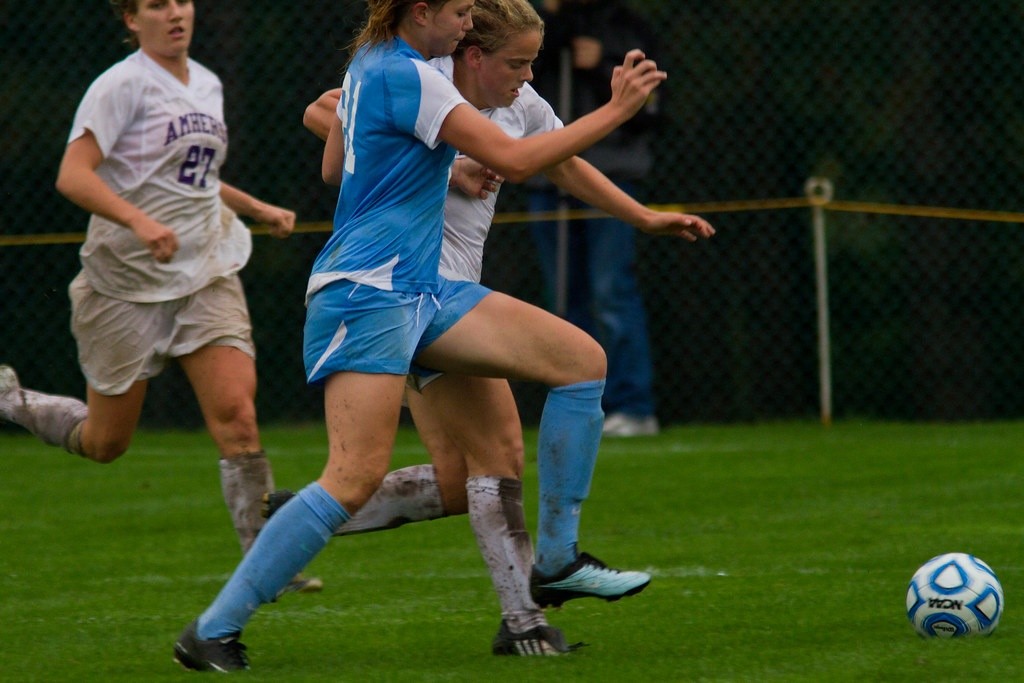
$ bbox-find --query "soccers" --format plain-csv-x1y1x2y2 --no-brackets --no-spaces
904,551,1004,638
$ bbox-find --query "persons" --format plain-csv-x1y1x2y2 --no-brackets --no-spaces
172,0,666,670
260,1,716,658
0,0,322,595
524,0,668,436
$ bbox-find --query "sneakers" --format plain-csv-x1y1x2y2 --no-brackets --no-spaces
174,618,250,672
529,552,651,608
492,618,591,657
262,490,295,518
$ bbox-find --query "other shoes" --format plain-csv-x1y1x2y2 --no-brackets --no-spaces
602,411,659,437
0,364,19,390
289,572,323,590
